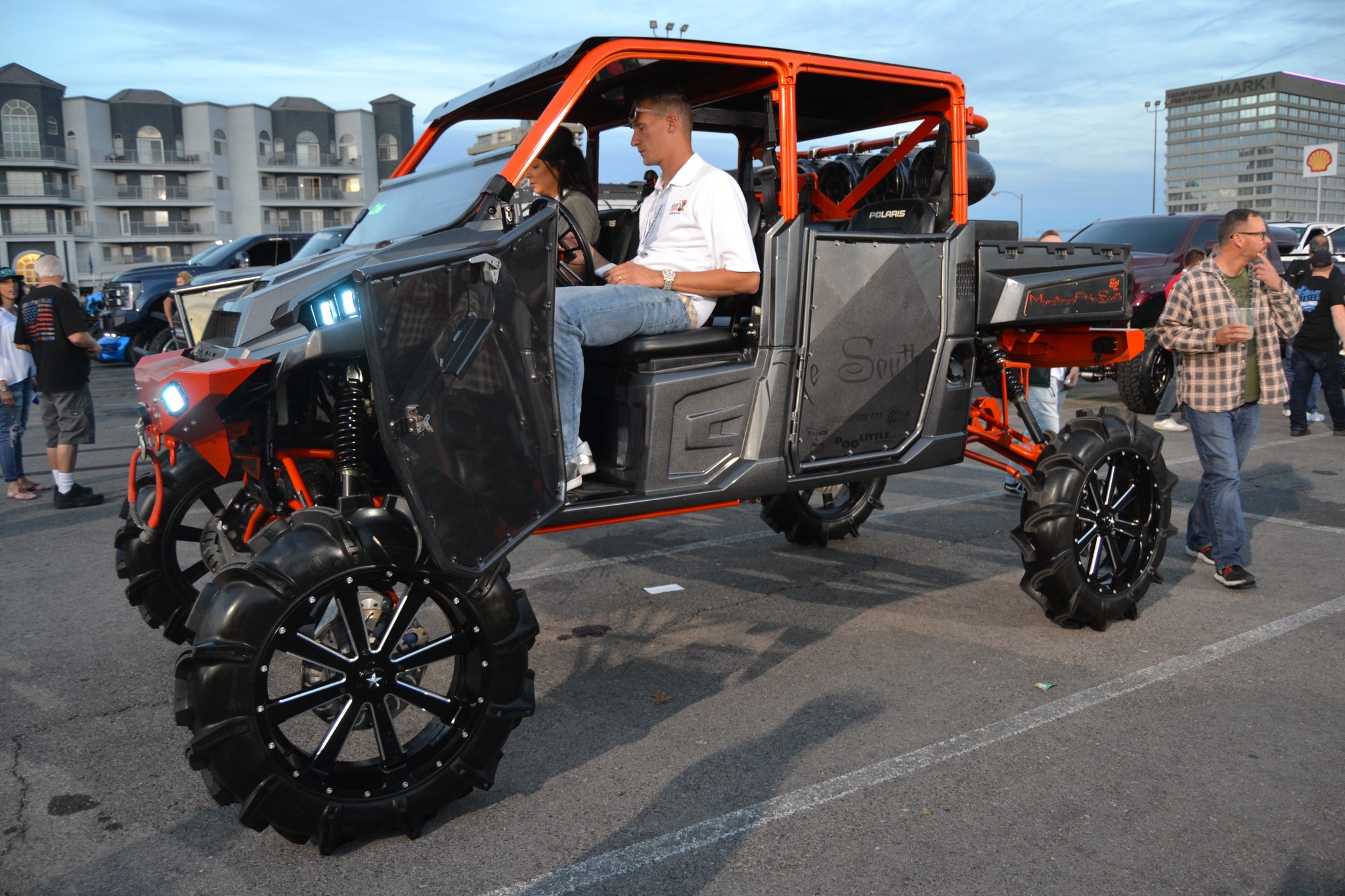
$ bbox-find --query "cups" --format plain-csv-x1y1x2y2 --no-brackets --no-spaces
1229,307,1256,340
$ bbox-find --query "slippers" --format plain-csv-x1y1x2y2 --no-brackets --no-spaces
6,487,37,500
26,482,50,491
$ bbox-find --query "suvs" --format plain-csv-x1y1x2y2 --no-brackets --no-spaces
93,36,1345,857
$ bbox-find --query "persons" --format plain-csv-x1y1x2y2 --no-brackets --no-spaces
0,266,54,501
1154,209,1305,587
1152,247,1207,432
1281,236,1345,422
1002,229,1081,498
525,126,602,286
552,81,764,494
13,254,106,509
163,270,194,350
1289,250,1345,437
14,278,89,310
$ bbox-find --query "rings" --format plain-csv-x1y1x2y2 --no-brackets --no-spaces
5,402,8,404
618,274,622,281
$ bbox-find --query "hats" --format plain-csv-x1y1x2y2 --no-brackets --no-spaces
1311,249,1332,268
0,266,24,283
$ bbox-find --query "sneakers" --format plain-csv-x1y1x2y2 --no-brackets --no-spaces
1305,411,1325,422
1333,428,1345,437
1003,482,1026,498
576,440,597,475
1282,409,1292,417
1153,417,1188,432
54,482,94,494
54,488,105,509
1185,545,1215,565
1214,564,1256,589
566,459,583,492
1290,428,1311,436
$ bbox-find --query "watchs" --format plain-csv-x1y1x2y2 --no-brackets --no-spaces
0,385,9,393
661,268,677,291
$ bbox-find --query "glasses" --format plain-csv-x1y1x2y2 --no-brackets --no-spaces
629,105,669,129
1229,232,1267,241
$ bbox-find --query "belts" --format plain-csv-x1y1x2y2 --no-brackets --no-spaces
676,292,698,330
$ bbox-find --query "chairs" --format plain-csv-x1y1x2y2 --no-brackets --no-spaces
584,193,767,369
